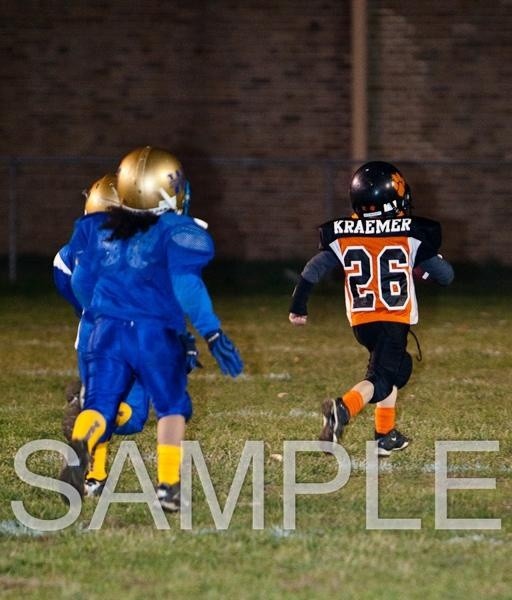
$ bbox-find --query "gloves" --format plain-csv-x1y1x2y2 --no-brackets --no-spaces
204,328,243,376
186,331,199,373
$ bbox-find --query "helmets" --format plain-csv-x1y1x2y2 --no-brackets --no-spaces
350,161,411,220
84,146,186,216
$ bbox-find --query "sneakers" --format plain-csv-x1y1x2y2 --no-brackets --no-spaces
59,439,108,508
375,428,409,455
156,481,180,511
319,397,351,455
62,393,80,441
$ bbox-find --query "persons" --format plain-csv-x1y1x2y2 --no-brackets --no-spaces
54,147,244,513
289,161,453,456
